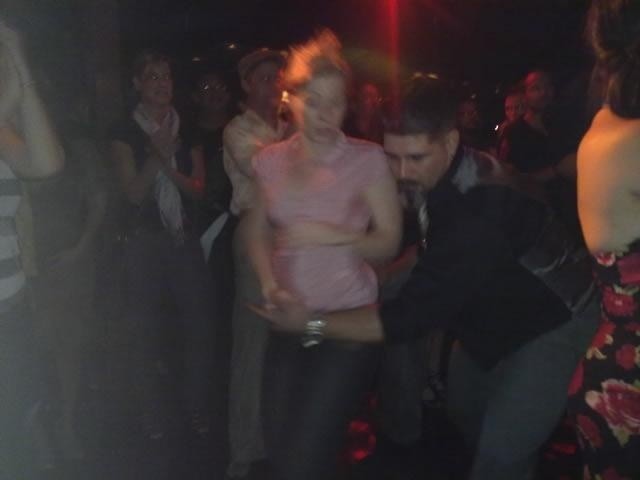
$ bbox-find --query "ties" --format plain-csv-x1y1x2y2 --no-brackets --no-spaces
413,198,429,244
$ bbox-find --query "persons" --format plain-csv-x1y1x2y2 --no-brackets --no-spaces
1,0,640,479
